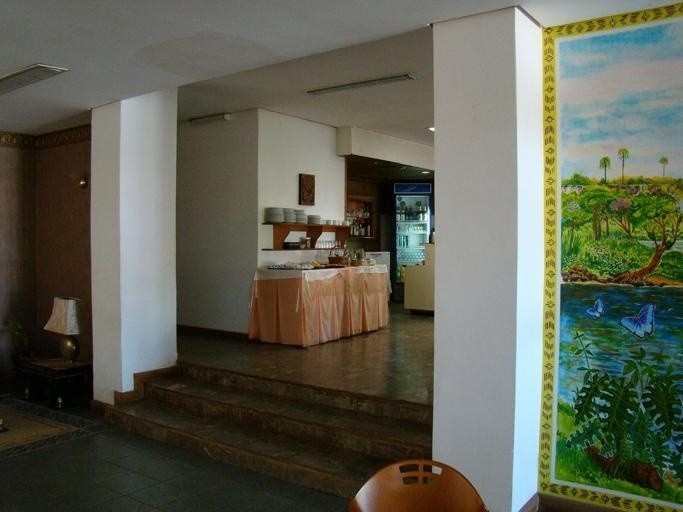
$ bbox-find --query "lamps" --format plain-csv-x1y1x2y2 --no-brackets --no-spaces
40,296,86,359
190,112,231,125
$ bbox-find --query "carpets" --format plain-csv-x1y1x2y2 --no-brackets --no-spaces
0,395,105,463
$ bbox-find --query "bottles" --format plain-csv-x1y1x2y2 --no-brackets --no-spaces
300,238,311,249
346,207,371,237
396,204,429,283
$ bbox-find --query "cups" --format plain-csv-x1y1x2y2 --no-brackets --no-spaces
314,241,342,249
326,220,349,226
348,247,365,267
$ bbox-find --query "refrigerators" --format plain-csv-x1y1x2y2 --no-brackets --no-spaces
391,183,432,302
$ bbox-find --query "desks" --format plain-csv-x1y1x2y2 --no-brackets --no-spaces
258,264,388,349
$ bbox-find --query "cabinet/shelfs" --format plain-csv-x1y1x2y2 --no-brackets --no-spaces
347,180,377,200
347,196,374,239
261,221,356,250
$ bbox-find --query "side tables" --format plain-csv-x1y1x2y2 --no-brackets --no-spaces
16,357,93,409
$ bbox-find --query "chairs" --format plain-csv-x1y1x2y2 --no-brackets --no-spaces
347,459,486,511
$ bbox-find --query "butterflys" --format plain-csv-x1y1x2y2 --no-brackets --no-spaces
619,302,656,339
586,298,607,318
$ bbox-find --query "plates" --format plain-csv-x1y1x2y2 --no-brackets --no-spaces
267,262,330,270
265,207,321,225
